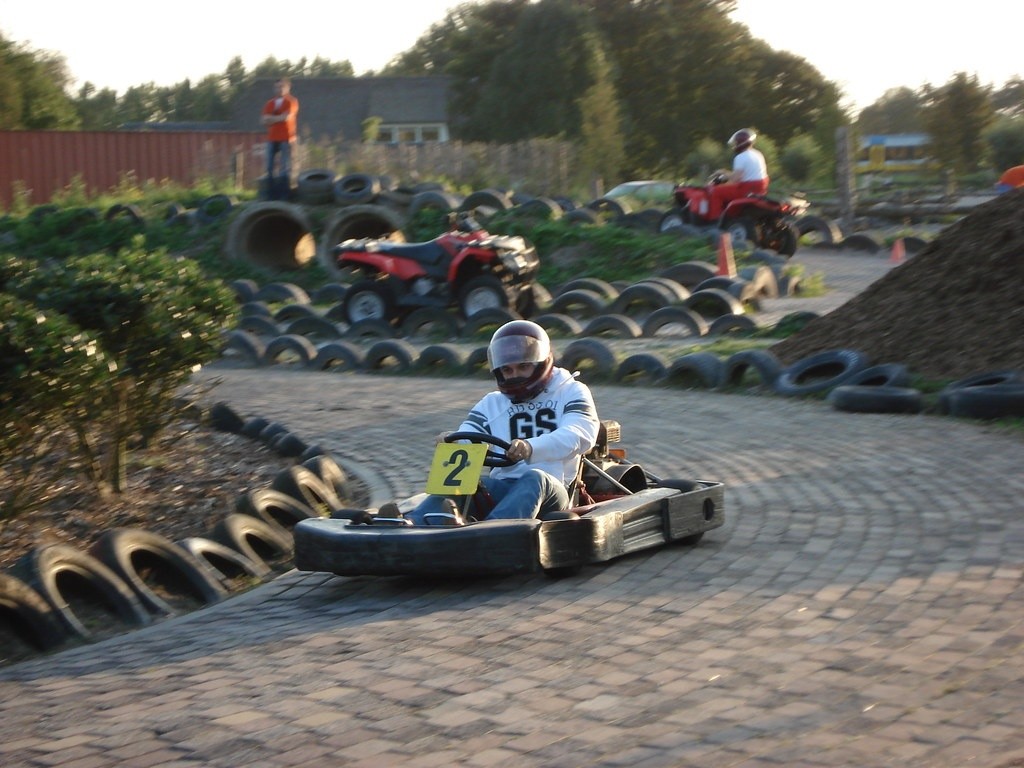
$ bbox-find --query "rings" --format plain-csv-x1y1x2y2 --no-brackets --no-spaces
518,454,523,457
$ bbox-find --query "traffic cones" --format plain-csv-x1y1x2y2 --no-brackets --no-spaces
714,232,737,279
886,238,908,265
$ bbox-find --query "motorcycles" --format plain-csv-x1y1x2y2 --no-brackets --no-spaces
319,203,540,327
657,168,812,259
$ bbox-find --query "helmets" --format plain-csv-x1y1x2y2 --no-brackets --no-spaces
734,129,753,152
490,319,553,404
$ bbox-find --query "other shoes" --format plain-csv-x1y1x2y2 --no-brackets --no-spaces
442,498,469,525
378,502,403,526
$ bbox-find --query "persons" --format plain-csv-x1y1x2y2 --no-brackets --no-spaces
260,77,299,180
377,320,600,525
696,128,769,225
